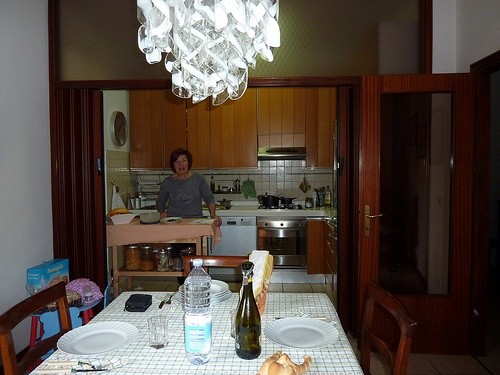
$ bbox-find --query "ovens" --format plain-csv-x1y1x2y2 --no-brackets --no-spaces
256,220,307,268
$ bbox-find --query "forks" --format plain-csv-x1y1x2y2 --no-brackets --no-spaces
165,291,176,304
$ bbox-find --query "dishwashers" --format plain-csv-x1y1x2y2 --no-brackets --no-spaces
207,216,257,256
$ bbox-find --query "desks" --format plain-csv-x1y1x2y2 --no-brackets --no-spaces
26,297,101,373
28,292,362,375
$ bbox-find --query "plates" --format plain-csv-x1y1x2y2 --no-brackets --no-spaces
57,321,139,357
173,280,233,306
263,317,339,350
160,216,182,224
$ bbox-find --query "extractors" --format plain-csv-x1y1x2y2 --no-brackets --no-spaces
257,147,306,161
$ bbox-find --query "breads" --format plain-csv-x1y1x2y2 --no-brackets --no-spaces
238,249,273,314
107,207,127,216
261,351,312,375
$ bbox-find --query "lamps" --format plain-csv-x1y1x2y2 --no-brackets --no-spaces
137,0,280,106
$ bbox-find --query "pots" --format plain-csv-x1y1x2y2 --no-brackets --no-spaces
257,192,279,206
280,197,297,204
220,198,232,209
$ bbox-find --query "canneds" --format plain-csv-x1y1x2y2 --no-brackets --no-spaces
222,186,228,192
125,244,193,272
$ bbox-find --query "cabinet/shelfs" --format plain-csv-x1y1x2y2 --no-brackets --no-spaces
106,217,221,298
128,85,338,171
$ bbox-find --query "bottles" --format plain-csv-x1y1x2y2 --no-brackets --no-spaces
182,258,213,366
125,243,194,272
234,178,240,194
234,260,262,360
210,176,215,193
305,185,330,208
84,285,93,302
126,192,146,210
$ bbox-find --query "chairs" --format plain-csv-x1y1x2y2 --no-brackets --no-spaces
183,255,251,291
0,286,73,375
359,282,418,375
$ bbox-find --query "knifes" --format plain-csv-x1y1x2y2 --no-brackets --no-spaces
158,293,170,309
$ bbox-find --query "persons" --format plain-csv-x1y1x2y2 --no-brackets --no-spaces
156,148,221,284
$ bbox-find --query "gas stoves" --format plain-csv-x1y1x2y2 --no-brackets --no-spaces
257,204,307,210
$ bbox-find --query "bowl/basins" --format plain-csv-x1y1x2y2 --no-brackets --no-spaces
140,212,160,224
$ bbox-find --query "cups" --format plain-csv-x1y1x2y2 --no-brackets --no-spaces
221,185,228,192
147,315,169,349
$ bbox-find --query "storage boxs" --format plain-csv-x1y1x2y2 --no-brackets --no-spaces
26,258,69,292
